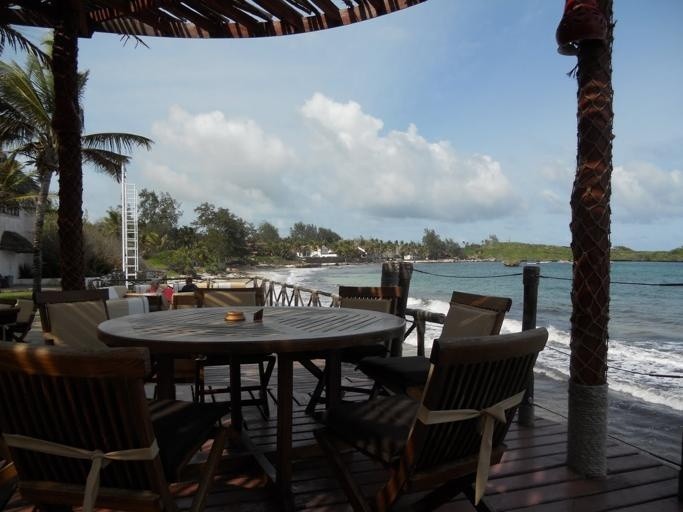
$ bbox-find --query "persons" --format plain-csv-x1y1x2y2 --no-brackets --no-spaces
145,279,160,293
182,277,199,291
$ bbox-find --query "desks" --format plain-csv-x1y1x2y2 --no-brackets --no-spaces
97,305,406,512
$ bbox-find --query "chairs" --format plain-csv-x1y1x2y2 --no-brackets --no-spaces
3,298,39,337
308,325,548,512
2,339,232,509
298,287,549,512
61,281,269,325
296,287,409,416
343,291,516,459
36,287,196,452
187,288,274,431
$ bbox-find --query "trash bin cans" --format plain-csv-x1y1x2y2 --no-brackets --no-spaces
6,275,13,288
